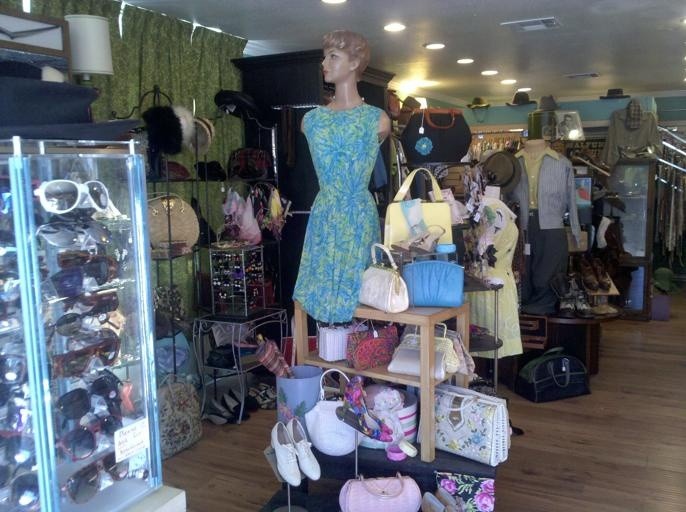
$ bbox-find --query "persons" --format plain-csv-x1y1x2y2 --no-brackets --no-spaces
291,30,390,323
513,139,582,316
561,115,580,136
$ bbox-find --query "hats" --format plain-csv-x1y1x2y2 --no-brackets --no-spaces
190,116,215,156
482,151,521,193
625,98,645,130
466,96,491,110
505,91,537,107
653,265,677,294
533,93,561,112
598,88,632,100
213,87,279,130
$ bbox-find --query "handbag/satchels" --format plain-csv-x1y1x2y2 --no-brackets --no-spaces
337,470,423,512
383,166,454,250
156,372,204,461
513,346,593,403
145,191,201,252
226,145,275,181
345,319,400,371
315,318,370,362
387,320,475,381
417,381,511,469
400,259,466,310
303,367,363,458
400,107,472,164
356,242,410,314
317,363,371,370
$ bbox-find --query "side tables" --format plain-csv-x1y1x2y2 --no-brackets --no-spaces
192,308,288,428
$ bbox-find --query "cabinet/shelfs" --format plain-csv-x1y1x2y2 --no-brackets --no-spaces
146,177,278,386
0,134,186,512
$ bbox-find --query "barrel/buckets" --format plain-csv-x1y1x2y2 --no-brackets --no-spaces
275,364,324,426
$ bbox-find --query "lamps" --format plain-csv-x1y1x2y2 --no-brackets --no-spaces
65,14,114,81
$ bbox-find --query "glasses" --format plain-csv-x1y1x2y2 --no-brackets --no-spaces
1,177,133,507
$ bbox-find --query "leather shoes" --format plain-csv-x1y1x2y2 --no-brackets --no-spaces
553,256,612,316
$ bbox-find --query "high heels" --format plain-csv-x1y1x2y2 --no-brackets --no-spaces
205,381,278,428
270,421,302,488
285,417,322,482
